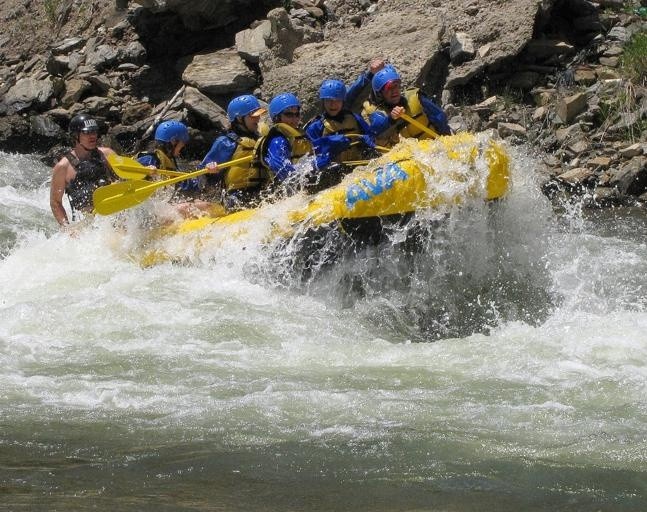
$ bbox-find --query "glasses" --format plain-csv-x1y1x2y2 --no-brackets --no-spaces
282,111,299,118
83,131,98,136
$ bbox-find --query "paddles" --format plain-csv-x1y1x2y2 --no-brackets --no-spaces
93,153,258,215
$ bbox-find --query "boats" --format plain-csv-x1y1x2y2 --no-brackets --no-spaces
128,131,511,274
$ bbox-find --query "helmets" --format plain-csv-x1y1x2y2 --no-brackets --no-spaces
371,67,401,96
69,114,100,135
319,79,346,99
154,120,191,143
268,92,301,119
227,95,267,123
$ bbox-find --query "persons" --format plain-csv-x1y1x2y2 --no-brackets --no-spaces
369,65,449,149
302,79,376,186
342,60,384,111
196,95,266,213
119,121,225,219
255,94,331,199
49,113,156,240
